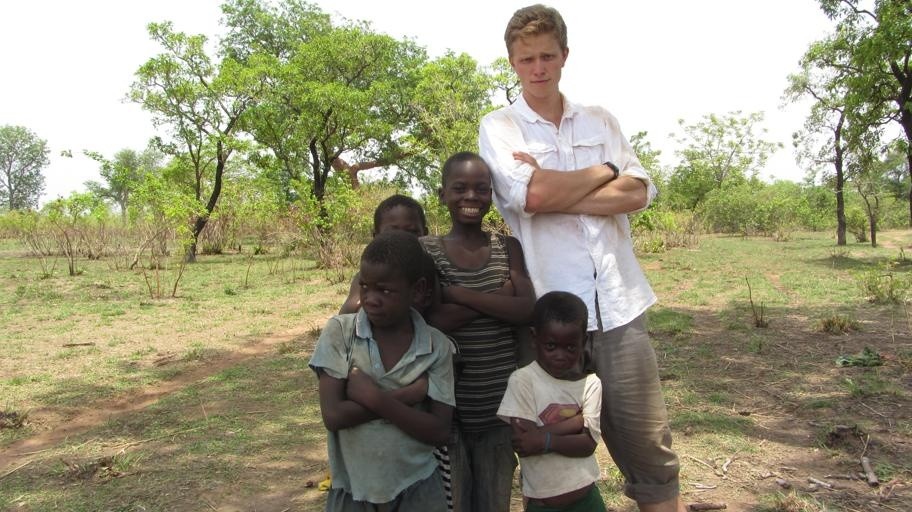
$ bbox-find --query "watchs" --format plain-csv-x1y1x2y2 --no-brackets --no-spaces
603,162,619,179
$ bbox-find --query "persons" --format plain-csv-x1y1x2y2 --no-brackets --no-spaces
338,194,429,314
478,4,686,512
418,152,537,512
307,230,456,512
496,291,605,512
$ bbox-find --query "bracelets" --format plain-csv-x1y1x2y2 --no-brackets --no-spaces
543,433,550,454
